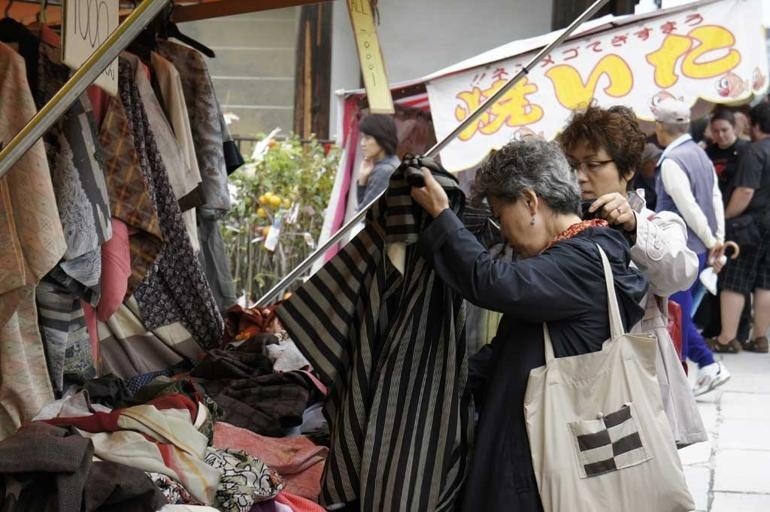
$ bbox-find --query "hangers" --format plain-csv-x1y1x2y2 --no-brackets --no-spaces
2,0,216,58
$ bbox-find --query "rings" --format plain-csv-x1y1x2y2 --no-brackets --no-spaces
616,207,623,213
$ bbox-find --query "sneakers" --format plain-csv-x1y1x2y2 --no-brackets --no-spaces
708,337,768,353
693,361,730,396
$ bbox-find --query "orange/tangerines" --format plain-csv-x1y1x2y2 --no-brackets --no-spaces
256,192,291,218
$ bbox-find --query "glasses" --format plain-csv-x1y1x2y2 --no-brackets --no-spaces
580,160,613,170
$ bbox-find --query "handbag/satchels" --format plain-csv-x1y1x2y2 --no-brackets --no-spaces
524,333,695,512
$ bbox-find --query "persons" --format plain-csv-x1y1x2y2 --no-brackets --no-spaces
559,98,710,448
408,134,650,512
355,112,402,214
641,98,769,395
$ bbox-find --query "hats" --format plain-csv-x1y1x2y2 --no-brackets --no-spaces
655,99,690,124
